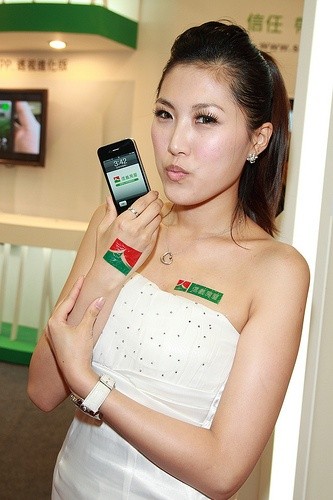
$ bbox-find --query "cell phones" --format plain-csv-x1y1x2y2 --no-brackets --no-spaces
97,139,152,216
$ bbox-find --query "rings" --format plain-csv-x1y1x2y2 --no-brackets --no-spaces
127,206,141,217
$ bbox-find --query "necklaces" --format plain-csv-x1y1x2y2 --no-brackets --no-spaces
160,212,245,266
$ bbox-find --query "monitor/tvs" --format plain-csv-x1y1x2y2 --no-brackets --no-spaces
0,88,48,166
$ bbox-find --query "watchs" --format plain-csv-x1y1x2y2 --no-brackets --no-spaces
71,375,116,420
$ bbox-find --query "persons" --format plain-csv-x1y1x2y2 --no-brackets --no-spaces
27,21,310,500
